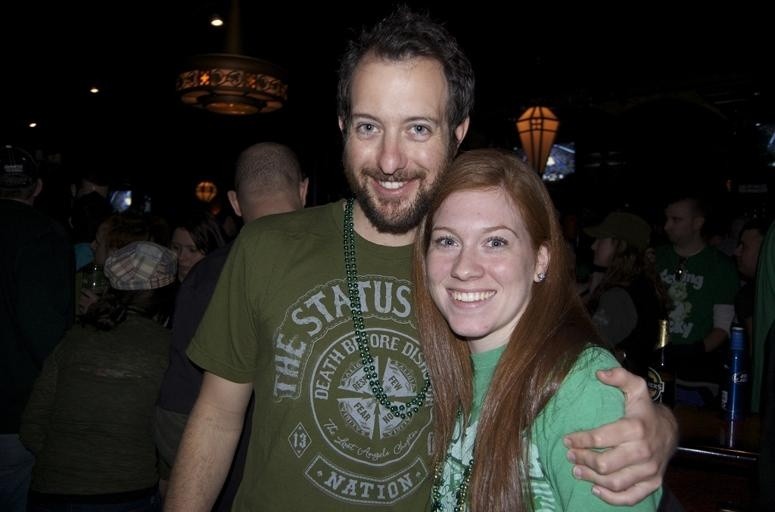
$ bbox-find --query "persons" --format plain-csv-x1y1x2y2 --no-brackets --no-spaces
414,149,663,512
556,192,774,428
163,7,680,512
1,141,310,511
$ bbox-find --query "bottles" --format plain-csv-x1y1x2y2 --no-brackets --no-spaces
648,315,753,423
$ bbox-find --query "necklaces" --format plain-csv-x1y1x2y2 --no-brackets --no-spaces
343,197,430,419
431,406,474,512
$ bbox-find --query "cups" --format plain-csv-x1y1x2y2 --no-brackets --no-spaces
82,262,109,296
